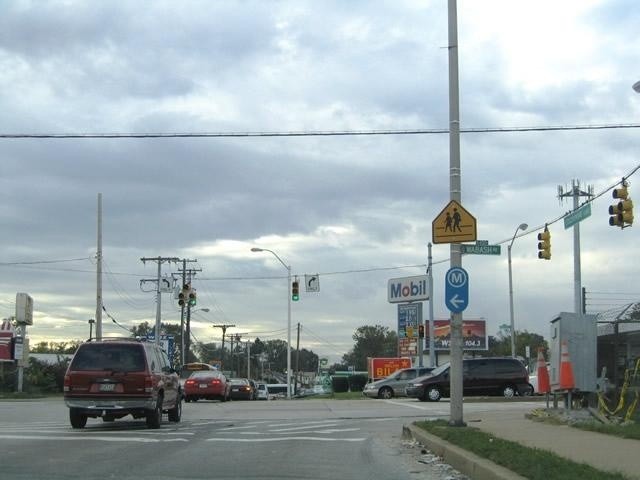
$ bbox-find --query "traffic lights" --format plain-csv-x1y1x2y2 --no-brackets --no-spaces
188,292,197,307
405,326,414,337
607,203,622,229
183,283,192,292
418,325,425,338
177,293,187,307
536,229,552,261
292,281,300,301
610,186,629,199
617,200,636,224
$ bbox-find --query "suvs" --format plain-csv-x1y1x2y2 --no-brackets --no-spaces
60,335,186,431
362,367,437,400
405,355,536,403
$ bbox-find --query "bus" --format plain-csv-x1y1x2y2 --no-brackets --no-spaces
265,383,302,399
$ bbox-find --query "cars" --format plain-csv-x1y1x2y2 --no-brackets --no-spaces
249,378,259,400
226,375,253,400
256,383,269,400
183,369,230,402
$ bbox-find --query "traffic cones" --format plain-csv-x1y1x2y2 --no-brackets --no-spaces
536,344,552,394
555,341,577,392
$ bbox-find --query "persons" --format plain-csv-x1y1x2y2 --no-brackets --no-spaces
451,207,463,233
442,210,453,234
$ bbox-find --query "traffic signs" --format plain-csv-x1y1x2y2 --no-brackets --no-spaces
564,203,592,231
460,244,501,255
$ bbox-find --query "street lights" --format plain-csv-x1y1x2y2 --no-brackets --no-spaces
190,308,211,316
250,245,294,399
508,221,530,358
